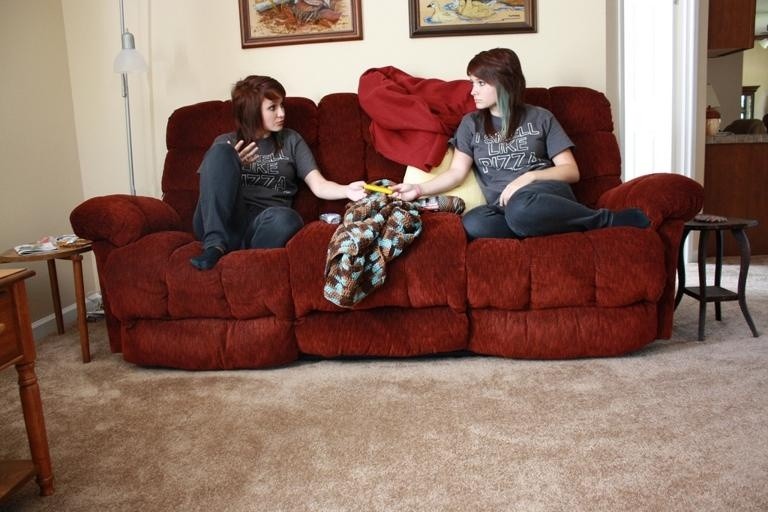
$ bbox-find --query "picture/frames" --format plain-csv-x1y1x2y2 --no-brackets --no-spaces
408,0,537,39
238,0,363,49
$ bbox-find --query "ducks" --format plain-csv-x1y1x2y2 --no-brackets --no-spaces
425,0,493,24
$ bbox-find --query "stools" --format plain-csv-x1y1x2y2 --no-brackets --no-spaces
673,218,760,341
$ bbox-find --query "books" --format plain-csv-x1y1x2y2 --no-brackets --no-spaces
12,240,59,256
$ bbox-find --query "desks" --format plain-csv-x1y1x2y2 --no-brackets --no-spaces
1,267,56,512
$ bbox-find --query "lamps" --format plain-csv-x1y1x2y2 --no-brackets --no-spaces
114,10,150,196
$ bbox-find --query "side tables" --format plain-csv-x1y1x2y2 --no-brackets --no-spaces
1,233,93,363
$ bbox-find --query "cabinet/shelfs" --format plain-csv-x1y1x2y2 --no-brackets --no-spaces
708,1,756,58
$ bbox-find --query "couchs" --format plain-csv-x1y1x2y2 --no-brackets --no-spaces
68,86,706,371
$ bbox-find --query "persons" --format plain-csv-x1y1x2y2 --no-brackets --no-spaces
384,47,650,242
183,72,370,271
